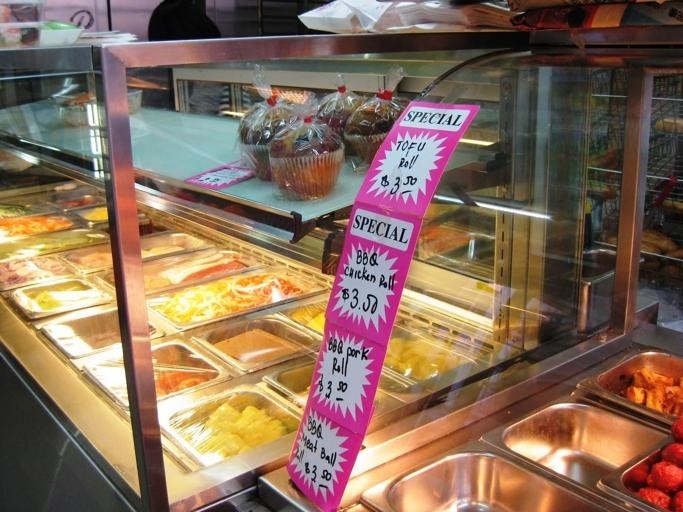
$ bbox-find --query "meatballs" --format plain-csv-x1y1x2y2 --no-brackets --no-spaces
629,415,683,512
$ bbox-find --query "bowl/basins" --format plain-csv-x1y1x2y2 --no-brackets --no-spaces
40,27,85,47
